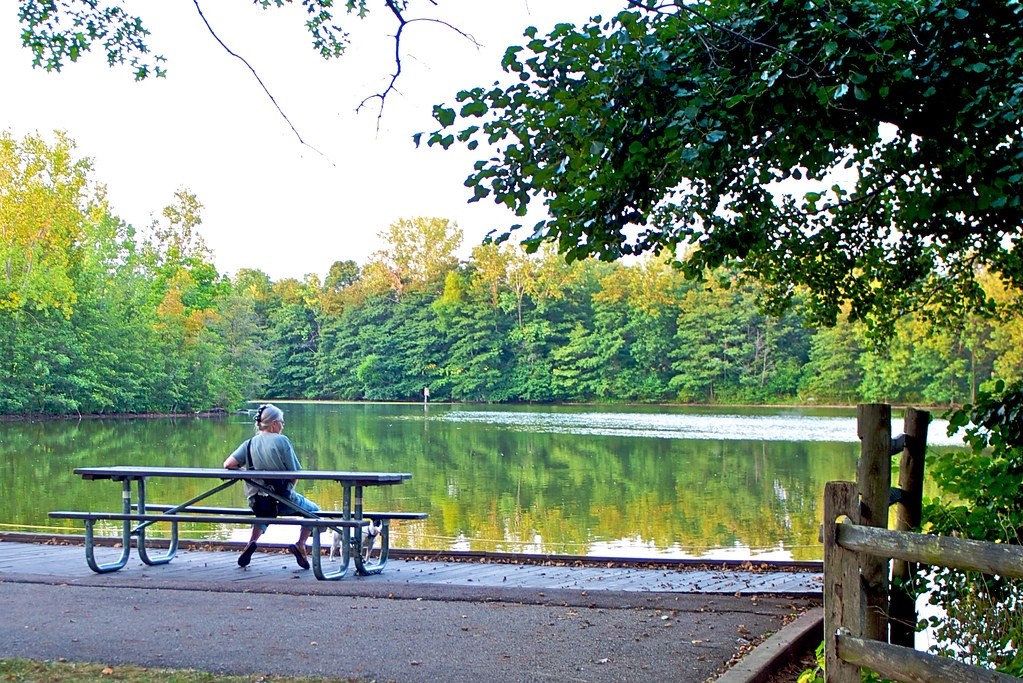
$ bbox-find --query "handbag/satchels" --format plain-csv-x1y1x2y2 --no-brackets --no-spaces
252,493,278,518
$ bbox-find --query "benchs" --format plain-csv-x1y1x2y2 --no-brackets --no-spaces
47,504,428,582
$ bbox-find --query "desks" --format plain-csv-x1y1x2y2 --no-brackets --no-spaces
73,465,412,582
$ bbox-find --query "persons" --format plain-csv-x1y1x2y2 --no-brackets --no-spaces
224,403,326,570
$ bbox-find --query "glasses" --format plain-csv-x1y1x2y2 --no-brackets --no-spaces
274,420,284,427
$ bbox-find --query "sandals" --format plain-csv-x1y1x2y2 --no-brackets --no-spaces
238,541,257,567
289,542,310,569
